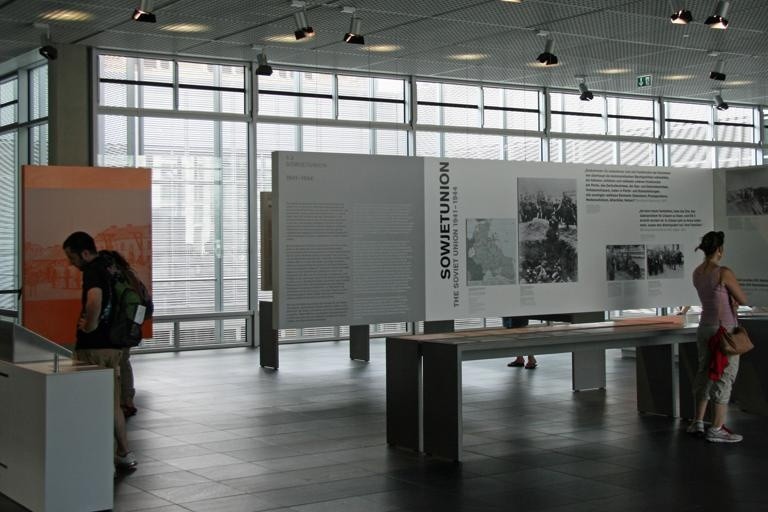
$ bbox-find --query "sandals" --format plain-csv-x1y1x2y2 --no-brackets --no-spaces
524,362,539,370
507,360,525,367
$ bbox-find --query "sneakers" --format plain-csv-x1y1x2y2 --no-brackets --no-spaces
114,463,121,480
121,403,137,419
118,449,138,472
705,424,745,445
685,419,706,434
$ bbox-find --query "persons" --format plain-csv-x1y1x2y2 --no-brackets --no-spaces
677,306,690,314
62,230,137,479
519,190,575,282
503,317,537,369
688,231,749,444
608,255,641,280
648,244,685,275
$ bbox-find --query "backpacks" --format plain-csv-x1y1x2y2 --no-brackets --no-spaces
98,249,155,347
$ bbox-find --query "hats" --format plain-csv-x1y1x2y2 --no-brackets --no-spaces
700,229,726,251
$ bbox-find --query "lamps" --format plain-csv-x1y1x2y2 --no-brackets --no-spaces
669,0,732,110
32,22,59,60
533,30,559,66
571,73,593,102
132,0,157,23
250,43,274,76
290,0,315,41
340,6,365,44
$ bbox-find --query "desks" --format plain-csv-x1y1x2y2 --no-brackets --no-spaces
383,310,767,468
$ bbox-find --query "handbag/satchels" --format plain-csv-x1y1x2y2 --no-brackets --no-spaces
719,324,756,356
